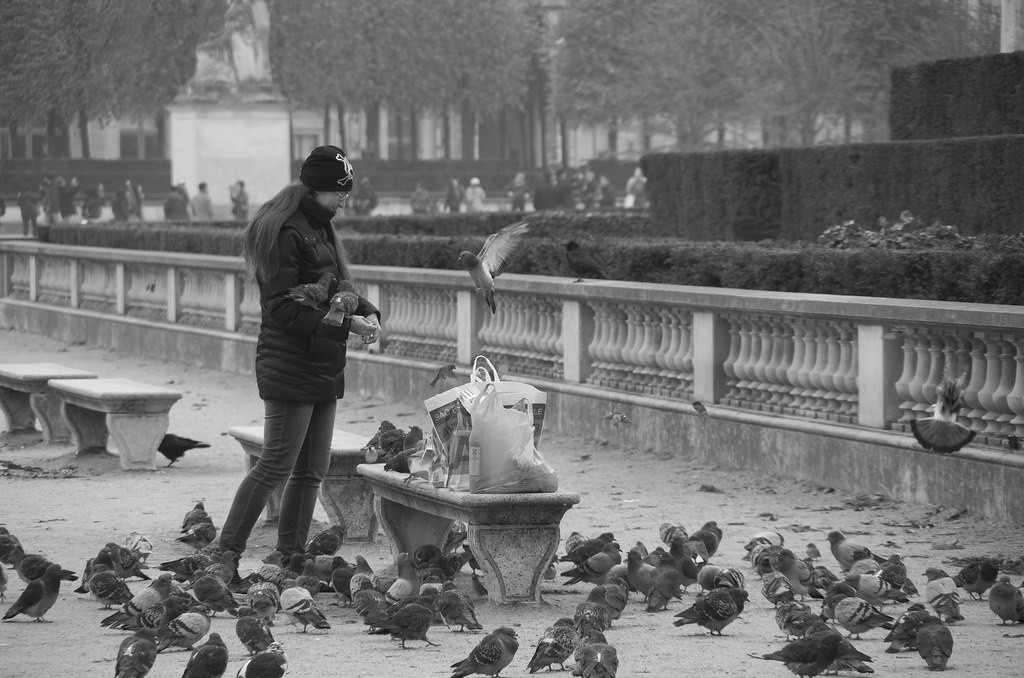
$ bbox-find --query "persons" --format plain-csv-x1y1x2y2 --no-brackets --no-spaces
18,165,648,240
205,147,383,598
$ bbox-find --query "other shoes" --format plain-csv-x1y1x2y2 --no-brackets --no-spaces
319,583,335,592
228,567,247,594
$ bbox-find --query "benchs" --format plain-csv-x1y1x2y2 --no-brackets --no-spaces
228,424,379,543
46,377,183,471
0,364,97,448
357,463,580,608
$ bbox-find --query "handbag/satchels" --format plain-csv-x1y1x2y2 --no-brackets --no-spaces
407,437,436,481
468,383,559,494
424,355,547,492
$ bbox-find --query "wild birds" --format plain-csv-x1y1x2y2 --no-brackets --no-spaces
600,412,632,429
743,530,1024,678
0,502,483,678
360,420,422,474
524,520,751,678
690,397,710,424
432,363,458,388
158,432,213,469
909,378,979,456
455,216,542,313
287,273,361,326
449,626,519,678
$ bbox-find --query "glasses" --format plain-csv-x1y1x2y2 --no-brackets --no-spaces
336,191,349,201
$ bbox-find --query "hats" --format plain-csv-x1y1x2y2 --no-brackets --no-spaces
299,145,354,191
